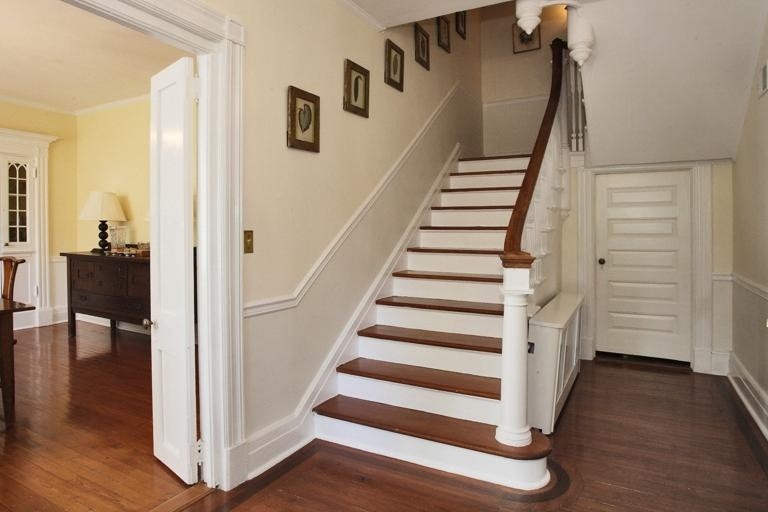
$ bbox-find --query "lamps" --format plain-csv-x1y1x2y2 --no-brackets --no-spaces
77,191,127,254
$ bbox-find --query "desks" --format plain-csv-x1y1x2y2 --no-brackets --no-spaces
0,294,35,425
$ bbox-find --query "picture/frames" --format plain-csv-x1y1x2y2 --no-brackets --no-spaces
513,23,541,54
287,11,466,153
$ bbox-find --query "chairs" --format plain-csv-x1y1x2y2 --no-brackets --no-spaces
0,256,26,387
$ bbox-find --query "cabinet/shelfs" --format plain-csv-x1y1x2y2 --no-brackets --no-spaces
526,293,585,435
60,247,197,337
